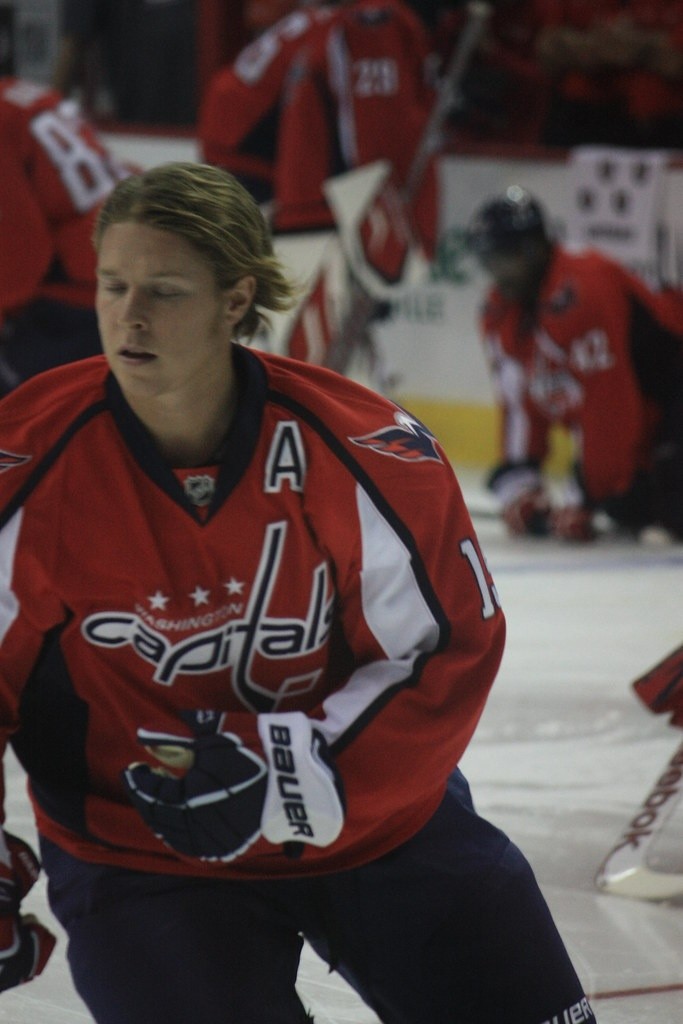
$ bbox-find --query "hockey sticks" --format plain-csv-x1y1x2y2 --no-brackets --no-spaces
589,732,683,904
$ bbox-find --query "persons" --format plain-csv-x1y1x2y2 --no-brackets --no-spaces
476,196,683,544
0,163,596,1024
59,0,682,369
0,76,145,396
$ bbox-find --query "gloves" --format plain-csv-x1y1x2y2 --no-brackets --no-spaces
486,462,550,533
549,462,598,540
0,831,57,993
122,710,342,863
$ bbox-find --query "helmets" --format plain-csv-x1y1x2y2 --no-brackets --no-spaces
469,188,540,255
